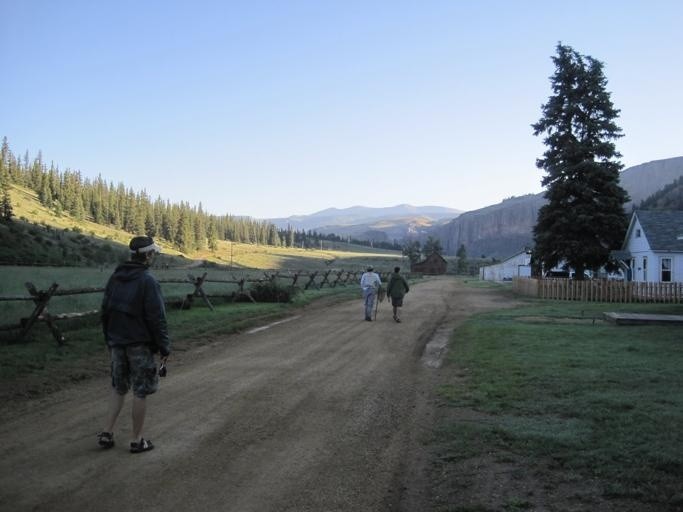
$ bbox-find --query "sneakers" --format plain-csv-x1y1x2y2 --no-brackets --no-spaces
97,432,114,448
129,439,154,453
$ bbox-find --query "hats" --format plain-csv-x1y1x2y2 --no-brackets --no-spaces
127,244,161,255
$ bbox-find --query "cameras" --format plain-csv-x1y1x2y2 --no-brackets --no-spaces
158,362,168,377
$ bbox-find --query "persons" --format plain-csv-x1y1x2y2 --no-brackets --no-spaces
387,266,410,322
96,235,172,453
360,264,382,322
